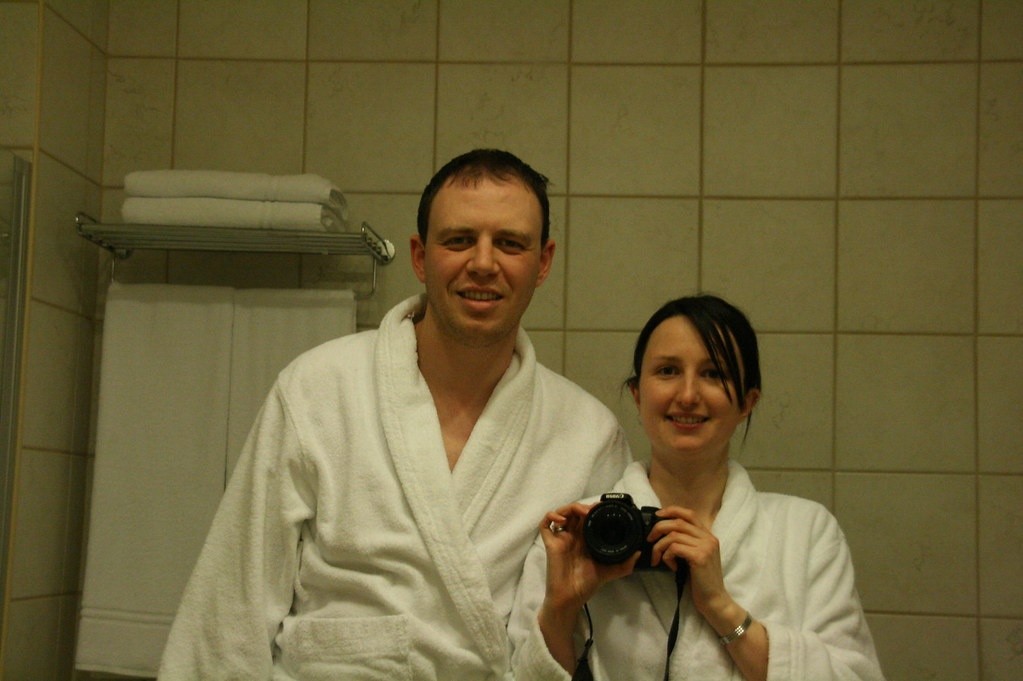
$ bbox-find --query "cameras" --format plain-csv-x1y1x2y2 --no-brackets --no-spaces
585,492,686,571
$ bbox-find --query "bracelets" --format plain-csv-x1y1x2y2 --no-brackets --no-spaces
719,612,753,646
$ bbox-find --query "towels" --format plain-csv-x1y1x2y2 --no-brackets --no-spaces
225,286,357,491
122,166,351,233
68,282,236,678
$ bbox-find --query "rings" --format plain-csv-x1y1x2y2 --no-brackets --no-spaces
553,525,568,533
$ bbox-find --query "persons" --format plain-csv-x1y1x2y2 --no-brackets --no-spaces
506,296,885,681
157,148,632,681
